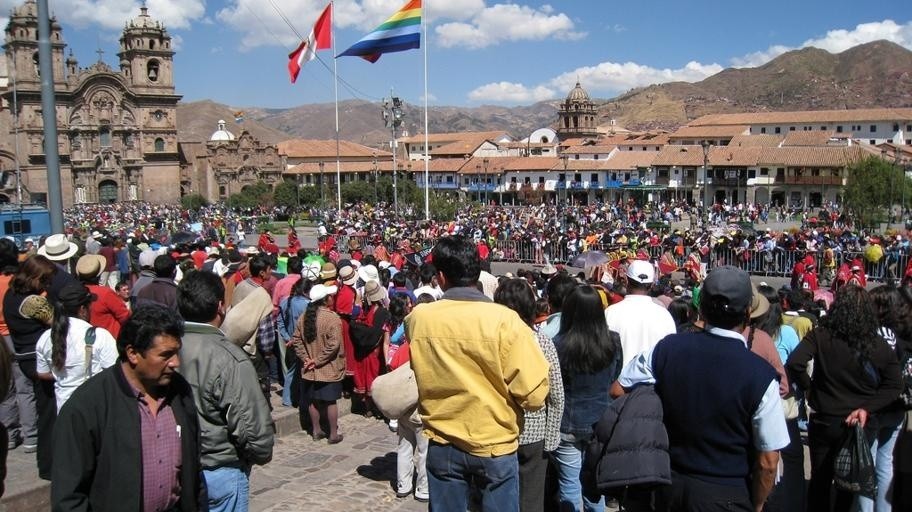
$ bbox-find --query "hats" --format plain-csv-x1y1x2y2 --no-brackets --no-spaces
701,265,752,312
379,260,392,269
849,266,862,272
37,234,79,261
783,231,788,237
301,260,322,280
749,280,770,319
58,281,97,306
541,263,557,274
80,233,88,241
337,265,359,285
806,246,818,253
91,231,103,238
805,263,815,271
358,265,379,283
623,259,655,284
245,246,259,254
319,264,337,280
76,254,106,279
309,283,338,302
23,238,33,243
337,259,356,272
762,234,771,238
364,280,385,305
349,259,361,270
824,233,831,240
207,246,219,257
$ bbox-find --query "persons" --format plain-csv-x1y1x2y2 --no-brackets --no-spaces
37,281,121,415
55,305,209,512
604,262,677,394
274,278,316,409
335,266,356,399
610,265,786,511
352,281,384,419
169,200,305,306
744,284,790,395
389,287,437,341
0,237,39,451
3,255,55,476
403,235,549,511
293,284,345,442
304,200,439,296
753,285,806,512
488,280,566,511
859,286,912,511
440,197,555,332
232,257,275,412
384,294,415,430
551,286,624,512
389,337,429,504
787,284,898,511
556,196,706,301
178,272,276,512
25,237,35,253
668,295,702,336
43,199,169,308
708,198,911,322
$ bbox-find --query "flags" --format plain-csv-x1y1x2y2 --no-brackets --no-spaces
233,110,247,123
334,0,422,60
286,0,332,86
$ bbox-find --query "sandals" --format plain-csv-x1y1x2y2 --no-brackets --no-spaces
327,435,344,444
312,431,327,442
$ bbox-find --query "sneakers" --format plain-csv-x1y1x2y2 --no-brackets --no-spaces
23,444,37,453
7,437,21,449
396,488,414,498
413,496,430,503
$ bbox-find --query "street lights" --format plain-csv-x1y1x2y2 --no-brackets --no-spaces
493,165,505,205
483,157,489,208
562,153,570,226
701,138,711,232
699,138,710,215
736,169,741,201
317,160,326,209
482,158,489,208
476,165,481,202
476,163,482,204
561,153,571,228
296,173,300,207
319,161,324,210
